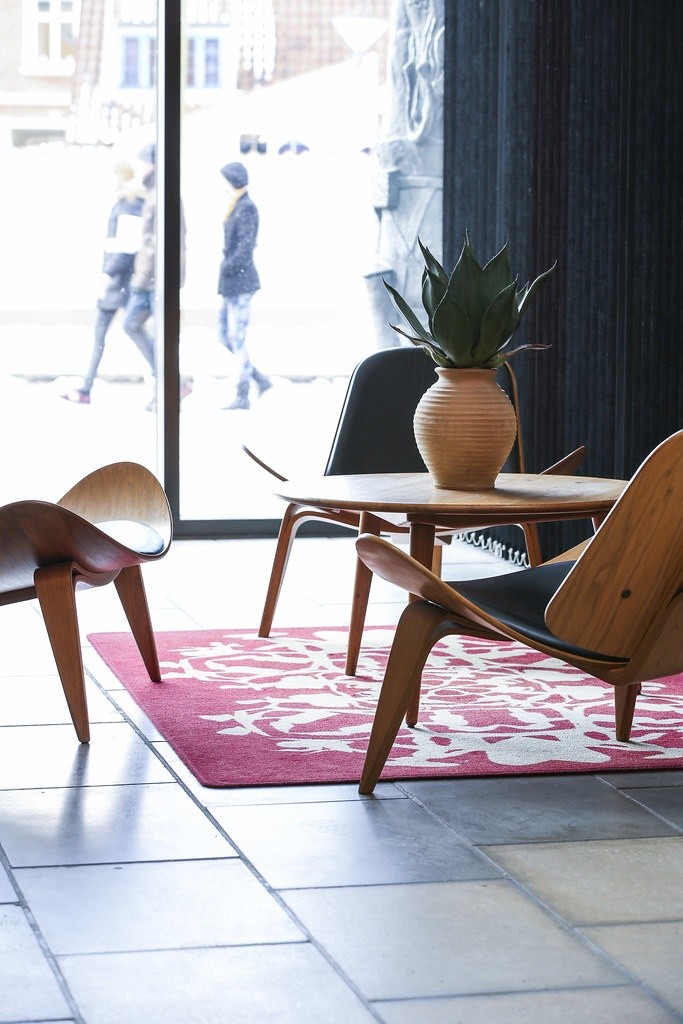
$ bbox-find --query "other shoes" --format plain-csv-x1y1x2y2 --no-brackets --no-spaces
256,377,273,401
179,374,194,403
145,397,157,413
61,388,91,404
221,396,251,410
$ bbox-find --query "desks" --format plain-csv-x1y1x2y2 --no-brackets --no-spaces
255,474,631,726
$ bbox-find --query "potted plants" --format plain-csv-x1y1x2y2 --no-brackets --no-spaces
381,226,560,489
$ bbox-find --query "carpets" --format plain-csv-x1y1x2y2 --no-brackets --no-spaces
86,626,683,789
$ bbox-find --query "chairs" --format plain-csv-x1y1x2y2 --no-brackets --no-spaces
0,462,172,744
241,347,585,677
356,430,683,796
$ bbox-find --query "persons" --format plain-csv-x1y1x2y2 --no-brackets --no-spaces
58,143,192,415
216,161,272,409
